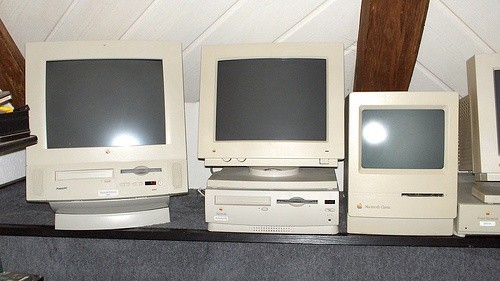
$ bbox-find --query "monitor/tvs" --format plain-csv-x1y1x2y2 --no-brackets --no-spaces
198,41,347,169
22,40,188,201
460,53,499,181
347,90,458,219
